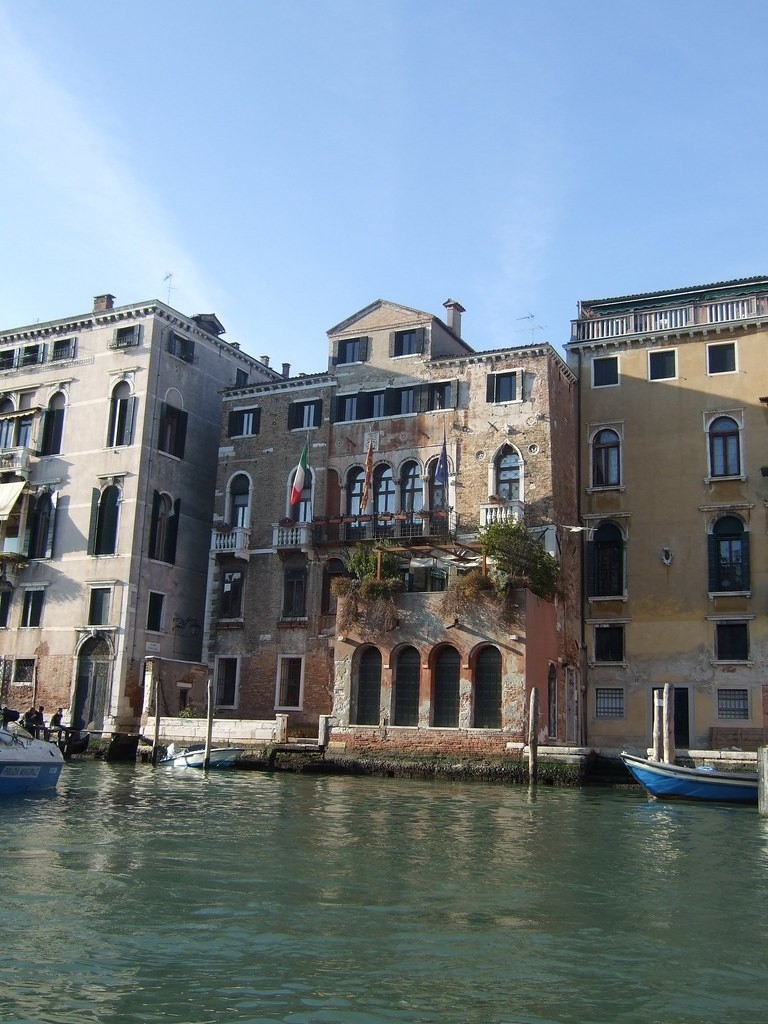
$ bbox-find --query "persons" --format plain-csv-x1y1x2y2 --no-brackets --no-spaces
50,709,69,746
21,707,36,737
33,706,49,741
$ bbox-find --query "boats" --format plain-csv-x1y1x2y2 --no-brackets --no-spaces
0,720,66,795
617,751,757,805
158,744,246,768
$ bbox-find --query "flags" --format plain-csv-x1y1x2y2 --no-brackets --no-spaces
435,421,448,489
290,438,308,505
359,428,374,509
